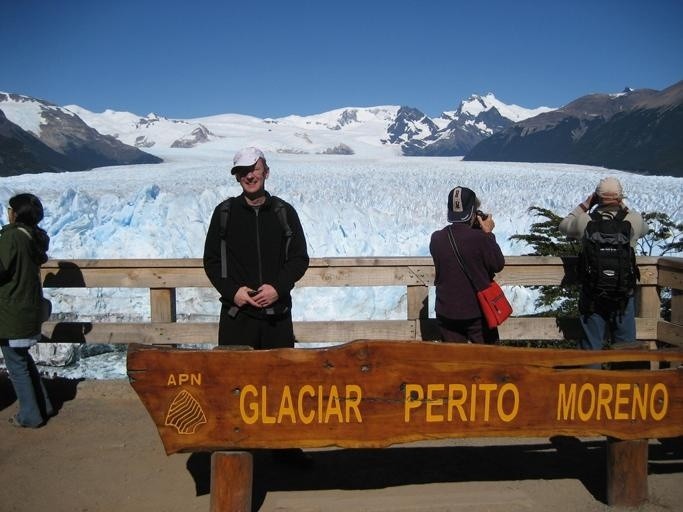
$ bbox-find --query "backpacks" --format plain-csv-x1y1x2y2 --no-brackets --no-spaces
580,210,637,315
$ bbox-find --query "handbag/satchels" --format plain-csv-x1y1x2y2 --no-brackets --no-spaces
478,281,513,331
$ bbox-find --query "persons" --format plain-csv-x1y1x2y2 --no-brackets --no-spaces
558,176,649,371
182,147,309,473
0,191,60,430
428,186,505,347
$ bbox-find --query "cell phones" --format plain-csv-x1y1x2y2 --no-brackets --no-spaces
248,291,259,297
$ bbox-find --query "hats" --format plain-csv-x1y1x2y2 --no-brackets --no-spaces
447,186,476,223
595,177,622,200
230,147,265,175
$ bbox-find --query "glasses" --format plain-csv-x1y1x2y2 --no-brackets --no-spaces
5,204,13,211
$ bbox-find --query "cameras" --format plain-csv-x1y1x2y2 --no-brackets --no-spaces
472,210,488,227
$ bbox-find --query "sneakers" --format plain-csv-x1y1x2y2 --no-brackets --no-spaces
9,410,56,428
